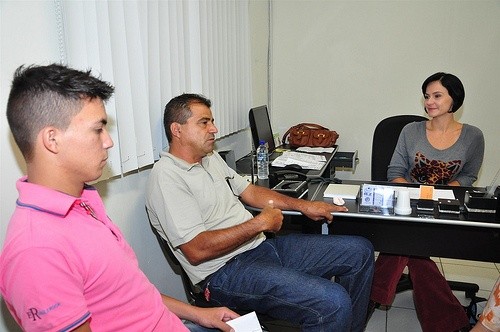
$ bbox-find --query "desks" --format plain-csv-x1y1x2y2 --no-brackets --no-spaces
237,144,500,263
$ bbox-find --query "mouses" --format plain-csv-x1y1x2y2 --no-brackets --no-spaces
282,164,302,172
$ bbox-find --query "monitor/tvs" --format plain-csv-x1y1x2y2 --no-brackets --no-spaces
248,105,279,160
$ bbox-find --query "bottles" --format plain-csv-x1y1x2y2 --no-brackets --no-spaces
257,140,269,179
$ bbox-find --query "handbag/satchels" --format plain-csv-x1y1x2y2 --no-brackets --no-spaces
282,123,339,148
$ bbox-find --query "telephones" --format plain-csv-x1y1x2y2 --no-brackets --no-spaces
269,170,311,185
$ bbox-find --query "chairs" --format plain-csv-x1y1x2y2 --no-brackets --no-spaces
370,114,479,299
160,237,302,332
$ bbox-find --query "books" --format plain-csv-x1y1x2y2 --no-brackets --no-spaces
323,183,361,200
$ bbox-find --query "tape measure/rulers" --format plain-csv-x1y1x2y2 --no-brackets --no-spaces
483,168,500,198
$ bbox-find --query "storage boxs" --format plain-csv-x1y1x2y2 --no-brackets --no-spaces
464,191,497,214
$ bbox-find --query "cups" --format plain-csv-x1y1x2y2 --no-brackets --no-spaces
394,190,411,215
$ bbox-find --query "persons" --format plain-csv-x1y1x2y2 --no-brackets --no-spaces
368,72,484,332
0,62,241,332
468,274,500,332
146,94,375,332
